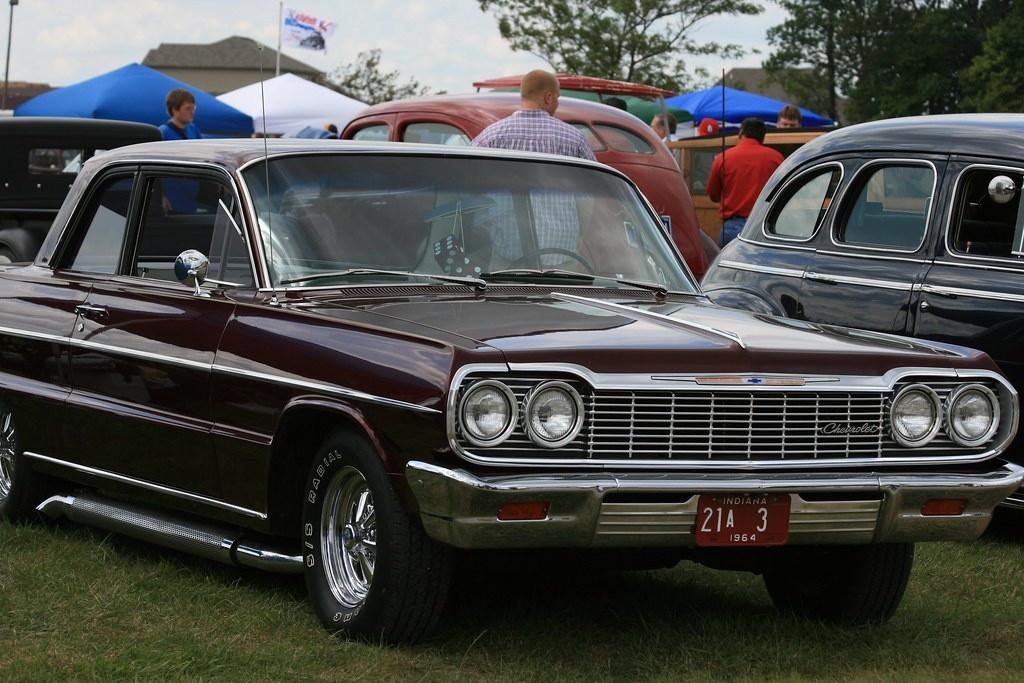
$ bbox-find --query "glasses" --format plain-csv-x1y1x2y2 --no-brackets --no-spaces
179,105,196,110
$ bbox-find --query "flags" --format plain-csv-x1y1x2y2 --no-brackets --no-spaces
280,7,337,51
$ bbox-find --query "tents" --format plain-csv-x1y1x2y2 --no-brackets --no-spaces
472,73,838,143
16,62,373,141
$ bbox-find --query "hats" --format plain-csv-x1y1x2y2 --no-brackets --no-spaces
698,118,719,137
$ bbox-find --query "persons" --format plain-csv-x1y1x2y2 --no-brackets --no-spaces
706,118,784,250
651,111,678,147
777,105,801,128
467,70,598,272
158,88,204,216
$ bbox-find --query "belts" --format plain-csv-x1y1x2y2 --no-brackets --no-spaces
724,216,748,221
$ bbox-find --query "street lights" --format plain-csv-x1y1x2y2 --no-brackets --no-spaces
4,0,18,109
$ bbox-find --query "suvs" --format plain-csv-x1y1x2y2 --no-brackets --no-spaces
0,114,261,264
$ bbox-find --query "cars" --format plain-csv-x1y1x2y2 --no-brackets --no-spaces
699,113,1024,512
664,126,933,247
0,138,1024,646
279,93,722,285
